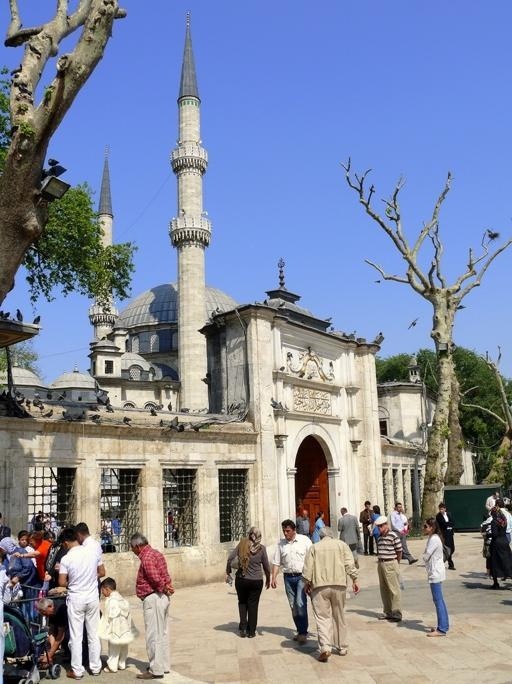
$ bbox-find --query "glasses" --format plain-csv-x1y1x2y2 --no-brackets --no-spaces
423,524,430,528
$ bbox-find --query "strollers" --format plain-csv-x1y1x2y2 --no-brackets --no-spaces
2,599,60,684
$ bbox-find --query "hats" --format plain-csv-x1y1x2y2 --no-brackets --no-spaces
374,515,388,526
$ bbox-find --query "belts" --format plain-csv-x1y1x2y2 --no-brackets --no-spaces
378,558,394,561
283,572,302,576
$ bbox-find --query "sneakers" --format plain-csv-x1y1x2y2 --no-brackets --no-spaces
408,559,418,564
426,627,446,636
233,628,247,637
391,617,401,621
318,650,331,661
296,634,308,644
67,670,84,680
247,629,257,637
448,566,456,570
136,671,164,679
336,648,349,655
104,665,119,673
146,667,169,673
293,631,308,640
378,612,393,619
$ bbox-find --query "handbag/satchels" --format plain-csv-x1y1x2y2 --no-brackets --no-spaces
443,544,451,561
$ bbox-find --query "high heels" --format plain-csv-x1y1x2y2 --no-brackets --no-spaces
490,582,500,590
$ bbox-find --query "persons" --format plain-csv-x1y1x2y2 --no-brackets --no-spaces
0,509,183,683
226,526,271,639
301,526,360,663
272,519,311,645
295,492,511,637
